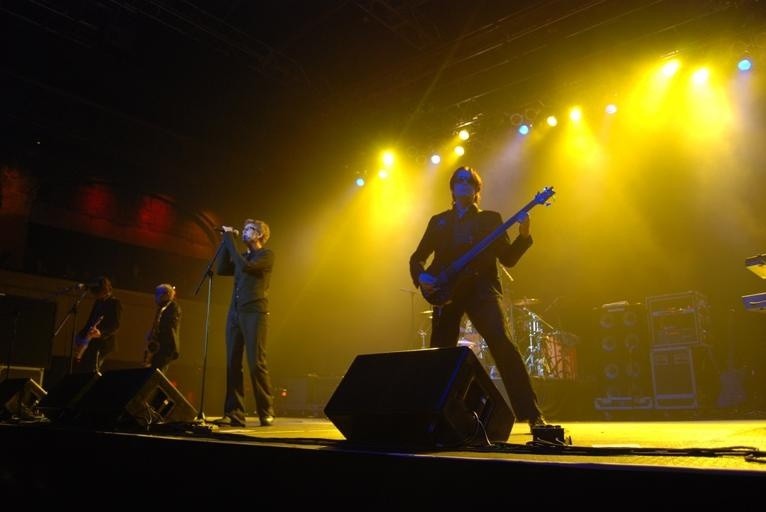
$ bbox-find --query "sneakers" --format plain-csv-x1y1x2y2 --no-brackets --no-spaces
260,415,275,426
529,416,547,433
214,416,236,424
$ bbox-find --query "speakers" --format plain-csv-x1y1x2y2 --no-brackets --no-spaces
324,345,515,453
37,372,103,431
76,366,199,435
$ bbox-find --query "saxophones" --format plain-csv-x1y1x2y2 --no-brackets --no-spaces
142,301,170,367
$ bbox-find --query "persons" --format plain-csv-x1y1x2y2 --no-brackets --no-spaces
148,283,181,373
79,278,122,376
410,165,549,435
215,219,275,426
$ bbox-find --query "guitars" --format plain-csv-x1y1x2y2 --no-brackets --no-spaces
421,186,557,306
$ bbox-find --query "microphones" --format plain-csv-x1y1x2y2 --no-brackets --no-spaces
215,227,240,237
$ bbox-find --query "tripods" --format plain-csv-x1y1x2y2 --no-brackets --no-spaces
520,313,548,378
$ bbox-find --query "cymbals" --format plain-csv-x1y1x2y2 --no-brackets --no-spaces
512,298,539,306
